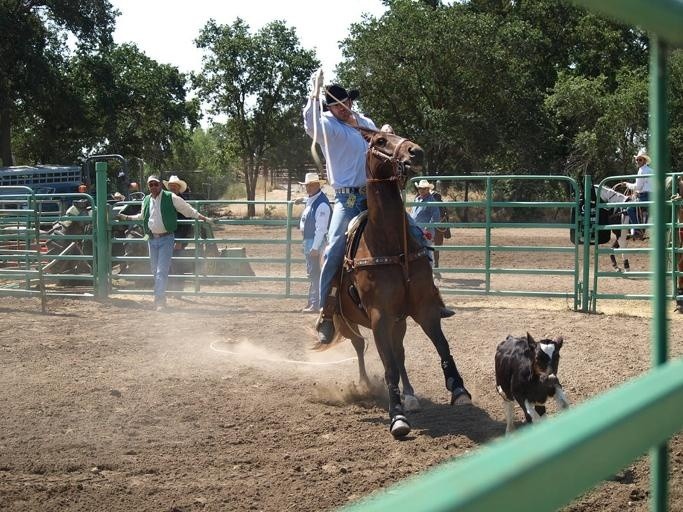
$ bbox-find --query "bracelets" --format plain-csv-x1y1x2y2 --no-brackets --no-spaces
125,215,128,221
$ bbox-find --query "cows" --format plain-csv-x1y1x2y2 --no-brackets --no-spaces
495,331,569,437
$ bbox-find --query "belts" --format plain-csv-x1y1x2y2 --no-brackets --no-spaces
152,232,169,238
334,186,367,195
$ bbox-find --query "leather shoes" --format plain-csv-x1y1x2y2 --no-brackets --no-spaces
441,307,456,318
317,319,334,343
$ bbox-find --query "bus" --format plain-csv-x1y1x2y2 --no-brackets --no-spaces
0,161,82,213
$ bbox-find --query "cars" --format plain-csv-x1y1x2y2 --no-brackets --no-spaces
0,226,48,266
23,182,81,215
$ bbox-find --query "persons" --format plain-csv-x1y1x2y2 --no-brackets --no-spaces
410,179,440,266
116,175,219,311
303,71,455,343
294,173,333,314
621,153,655,238
163,175,188,298
111,192,125,274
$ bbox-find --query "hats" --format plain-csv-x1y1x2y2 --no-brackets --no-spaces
298,173,326,185
322,85,360,106
163,175,187,194
110,192,126,201
147,175,160,183
414,179,435,190
633,149,651,165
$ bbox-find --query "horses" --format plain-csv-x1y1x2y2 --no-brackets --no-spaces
301,124,473,436
571,184,648,272
431,193,451,280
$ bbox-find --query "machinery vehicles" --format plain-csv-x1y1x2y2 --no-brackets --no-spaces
48,151,257,288
